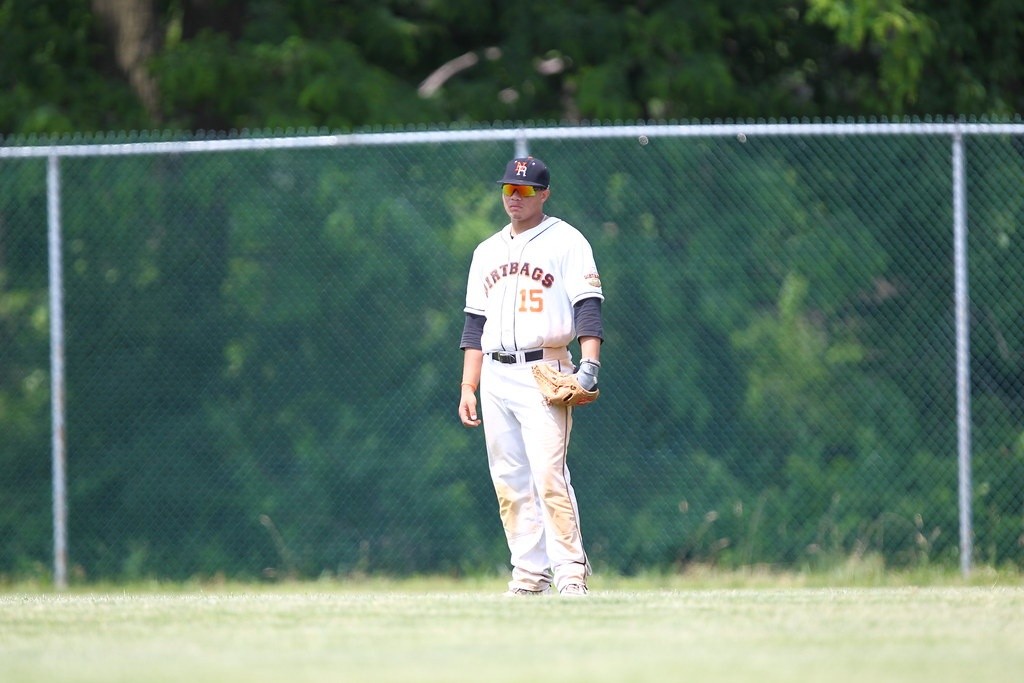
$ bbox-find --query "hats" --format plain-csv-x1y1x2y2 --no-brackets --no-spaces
496,157,550,190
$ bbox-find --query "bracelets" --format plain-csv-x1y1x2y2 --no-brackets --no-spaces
580,358,601,367
461,382,477,390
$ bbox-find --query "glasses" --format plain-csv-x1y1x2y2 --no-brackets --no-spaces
500,183,545,197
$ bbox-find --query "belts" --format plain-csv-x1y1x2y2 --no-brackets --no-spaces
487,345,569,364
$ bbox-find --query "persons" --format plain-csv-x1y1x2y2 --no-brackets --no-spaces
459,156,605,595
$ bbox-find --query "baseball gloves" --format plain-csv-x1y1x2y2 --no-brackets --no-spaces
534,362,600,408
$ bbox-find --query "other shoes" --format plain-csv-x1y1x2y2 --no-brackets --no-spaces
560,584,588,596
502,585,552,597
